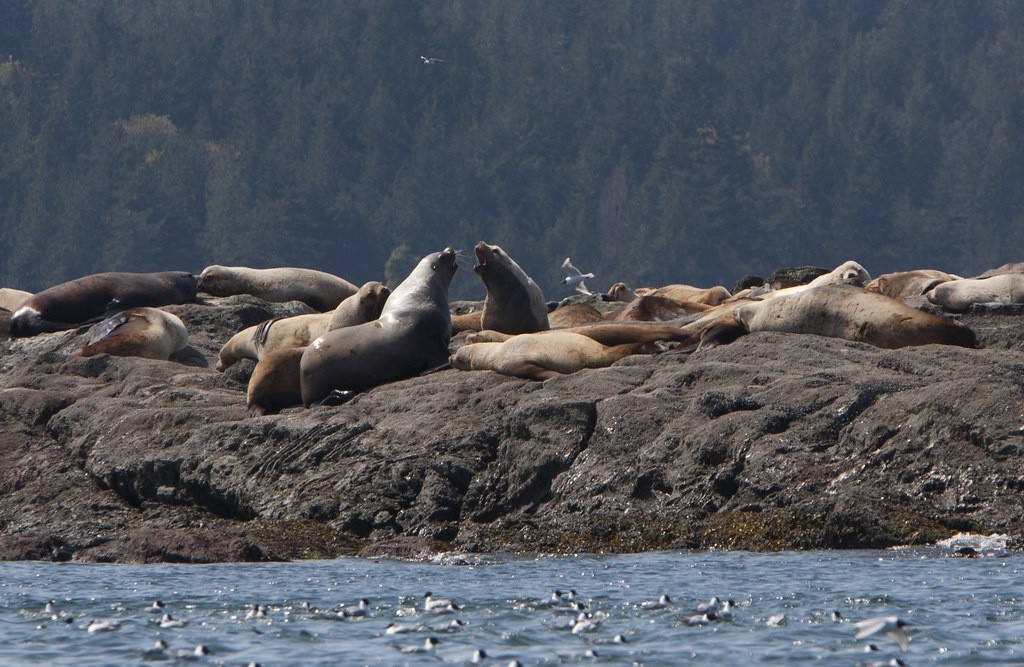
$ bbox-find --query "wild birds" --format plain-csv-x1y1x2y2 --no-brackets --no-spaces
36,585,918,667
558,256,599,299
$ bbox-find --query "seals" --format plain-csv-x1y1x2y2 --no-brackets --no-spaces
0,237,1024,414
